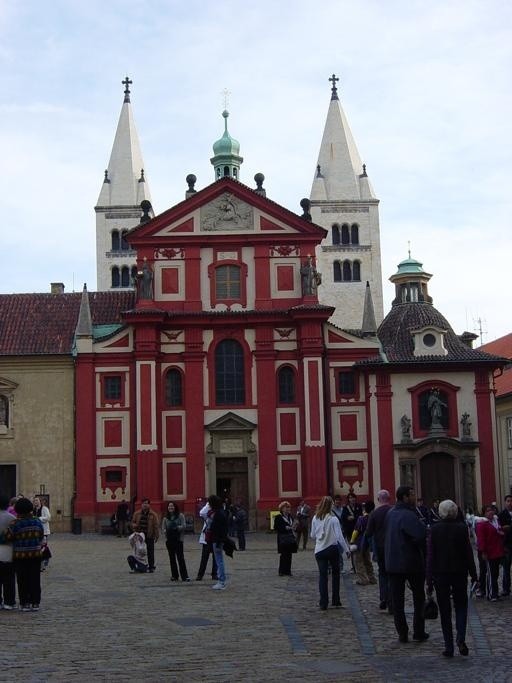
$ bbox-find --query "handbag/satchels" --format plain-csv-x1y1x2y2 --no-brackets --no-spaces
277,533,297,553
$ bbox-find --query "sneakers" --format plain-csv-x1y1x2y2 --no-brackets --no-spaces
332,602,342,606
212,582,225,589
470,581,512,603
130,567,155,573
171,576,178,581
442,650,454,658
456,638,468,655
0,599,39,612
182,577,191,582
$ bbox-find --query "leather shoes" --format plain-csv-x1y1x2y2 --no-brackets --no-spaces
212,575,219,579
279,571,293,576
196,576,202,581
356,575,429,642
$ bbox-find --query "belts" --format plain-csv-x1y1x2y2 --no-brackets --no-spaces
433,519,440,521
419,517,426,521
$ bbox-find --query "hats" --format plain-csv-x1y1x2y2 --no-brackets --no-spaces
432,498,441,503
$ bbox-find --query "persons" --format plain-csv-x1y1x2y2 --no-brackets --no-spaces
136,260,154,301
0,498,45,612
274,500,299,577
162,501,191,584
192,490,249,592
310,488,511,612
130,497,160,574
117,498,129,538
425,496,478,658
126,531,151,574
110,511,120,536
0,492,19,611
384,486,430,643
300,255,322,296
294,498,311,552
6,496,18,519
30,495,52,572
427,390,448,425
